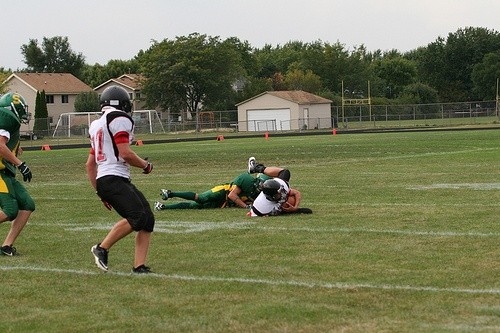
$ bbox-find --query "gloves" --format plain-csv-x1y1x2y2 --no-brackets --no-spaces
18,162,32,182
96,191,112,211
246,204,253,209
142,162,153,174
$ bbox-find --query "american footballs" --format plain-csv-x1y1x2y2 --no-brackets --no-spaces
281,197,294,211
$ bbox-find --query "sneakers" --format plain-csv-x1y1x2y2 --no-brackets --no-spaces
91,243,109,271
131,264,155,274
0,244,20,257
248,156,264,173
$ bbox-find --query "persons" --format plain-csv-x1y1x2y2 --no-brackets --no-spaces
0,92,36,256
86,84,153,274
156,156,312,219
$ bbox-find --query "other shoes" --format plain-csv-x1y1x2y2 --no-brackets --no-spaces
159,189,173,200
153,200,165,211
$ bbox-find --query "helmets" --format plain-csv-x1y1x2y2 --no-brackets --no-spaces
254,172,273,194
263,179,288,203
100,85,131,112
0,93,30,123
